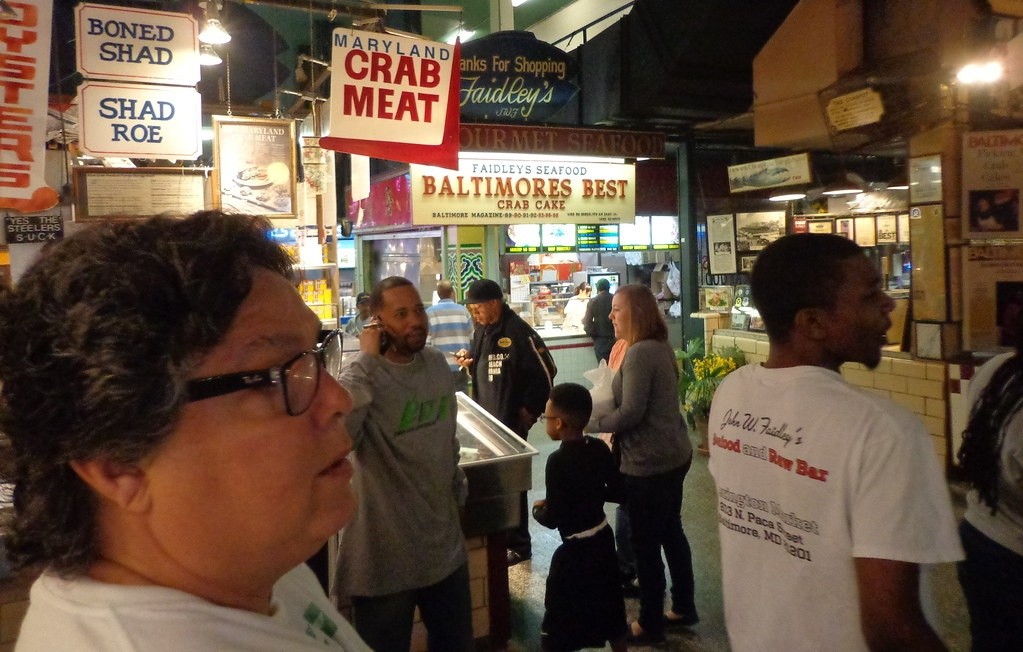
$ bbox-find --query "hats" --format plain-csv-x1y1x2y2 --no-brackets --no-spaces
457,279,503,305
357,292,370,303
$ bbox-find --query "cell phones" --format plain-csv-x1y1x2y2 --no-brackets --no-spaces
449,351,461,359
379,331,396,355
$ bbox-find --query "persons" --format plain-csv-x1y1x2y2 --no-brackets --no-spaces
956,349,1023,652
532,279,698,652
344,293,373,339
453,278,557,566
709,232,965,652
424,282,475,395
0,211,374,652
330,277,473,652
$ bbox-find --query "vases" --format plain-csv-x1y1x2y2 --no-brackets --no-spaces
694,414,711,456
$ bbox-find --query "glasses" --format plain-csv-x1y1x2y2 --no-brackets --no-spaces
184,328,343,417
540,414,566,427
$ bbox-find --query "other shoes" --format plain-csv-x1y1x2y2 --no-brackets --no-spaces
623,575,642,594
664,610,699,626
626,622,666,643
507,550,532,566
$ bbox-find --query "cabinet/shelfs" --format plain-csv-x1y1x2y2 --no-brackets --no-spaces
294,262,342,332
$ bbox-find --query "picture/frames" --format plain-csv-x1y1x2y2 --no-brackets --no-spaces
211,113,298,220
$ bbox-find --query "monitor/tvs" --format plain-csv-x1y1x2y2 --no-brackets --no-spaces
587,272,620,298
499,213,680,255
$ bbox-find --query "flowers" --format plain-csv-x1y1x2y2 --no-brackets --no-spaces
674,337,750,430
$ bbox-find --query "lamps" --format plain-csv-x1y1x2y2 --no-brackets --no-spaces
199,41,222,66
197,0,232,44
337,217,353,238
295,54,327,83
